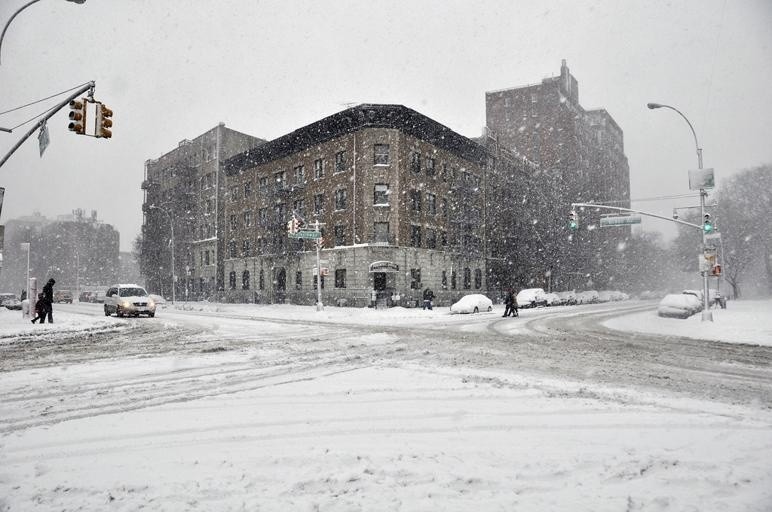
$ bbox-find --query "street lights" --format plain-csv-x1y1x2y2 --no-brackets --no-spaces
647,103,714,322
148,204,175,302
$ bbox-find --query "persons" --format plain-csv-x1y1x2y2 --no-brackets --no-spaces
369,285,437,311
501,287,519,317
40,277,56,323
31,293,44,325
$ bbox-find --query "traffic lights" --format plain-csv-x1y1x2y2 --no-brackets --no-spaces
96,104,113,138
285,214,302,234
704,213,711,233
568,211,579,231
713,264,722,275
67,98,85,135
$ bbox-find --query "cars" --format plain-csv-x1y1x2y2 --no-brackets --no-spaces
450,294,493,314
658,288,715,320
515,288,653,309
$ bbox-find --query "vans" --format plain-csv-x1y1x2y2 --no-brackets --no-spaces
1,292,22,310
87,290,105,303
53,289,73,304
78,290,91,302
104,283,156,318
